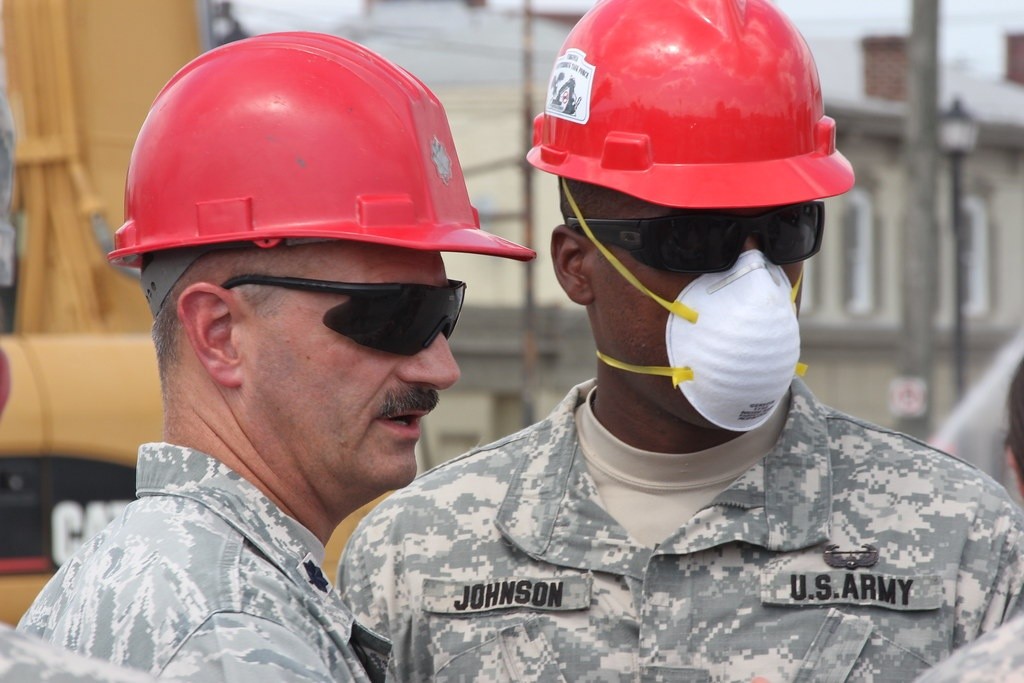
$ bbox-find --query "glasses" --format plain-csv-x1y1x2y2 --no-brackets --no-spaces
566,201,827,276
220,271,466,355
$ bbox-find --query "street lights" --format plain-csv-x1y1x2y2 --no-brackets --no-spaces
935,98,979,407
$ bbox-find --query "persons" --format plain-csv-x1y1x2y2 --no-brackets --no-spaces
1,357,158,682
14,30,536,683
913,356,1024,681
338,1,1024,681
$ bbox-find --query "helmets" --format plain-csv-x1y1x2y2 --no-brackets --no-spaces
106,30,537,266
526,1,856,209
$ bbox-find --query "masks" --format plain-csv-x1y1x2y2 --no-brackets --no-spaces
561,174,809,431
